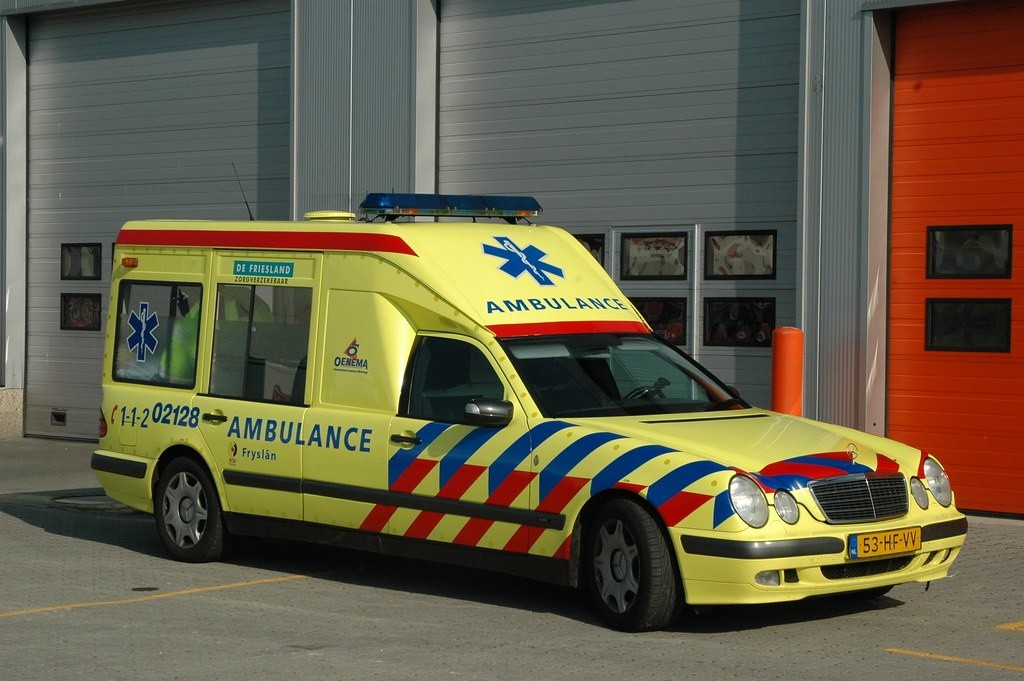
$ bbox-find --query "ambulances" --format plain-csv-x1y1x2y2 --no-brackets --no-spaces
90,193,970,632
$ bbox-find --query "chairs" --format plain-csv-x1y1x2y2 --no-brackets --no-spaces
421,360,484,420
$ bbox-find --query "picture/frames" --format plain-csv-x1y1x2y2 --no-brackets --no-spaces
704,229,777,280
620,232,688,280
61,293,101,331
925,224,1013,279
571,233,605,268
628,297,687,346
926,298,1011,352
704,296,776,348
60,243,101,280
